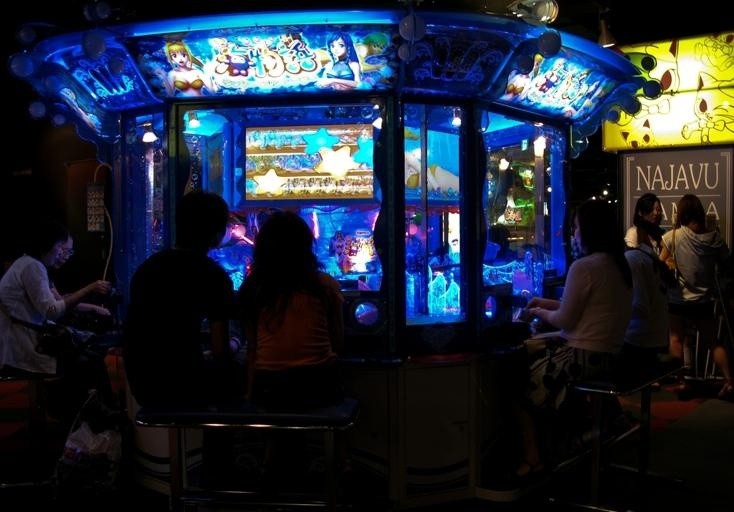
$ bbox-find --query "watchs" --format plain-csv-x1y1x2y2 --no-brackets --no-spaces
533,306,541,317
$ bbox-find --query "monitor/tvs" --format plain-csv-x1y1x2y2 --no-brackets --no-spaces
402,120,463,201
246,124,374,201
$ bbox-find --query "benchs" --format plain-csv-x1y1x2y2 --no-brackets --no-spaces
2,364,58,489
136,396,362,511
546,352,682,512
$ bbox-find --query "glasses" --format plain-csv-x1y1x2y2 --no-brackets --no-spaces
63,248,74,255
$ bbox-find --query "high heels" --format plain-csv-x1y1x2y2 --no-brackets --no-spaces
716,378,734,400
662,380,695,396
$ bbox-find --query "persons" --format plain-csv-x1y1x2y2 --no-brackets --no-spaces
234,211,360,483
622,194,672,391
596,244,667,444
511,200,630,473
123,188,251,461
46,231,119,436
489,222,511,255
0,227,113,431
658,194,734,397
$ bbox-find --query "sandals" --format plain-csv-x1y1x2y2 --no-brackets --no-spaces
513,461,546,478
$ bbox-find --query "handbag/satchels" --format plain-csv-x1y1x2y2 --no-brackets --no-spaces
43,328,98,373
